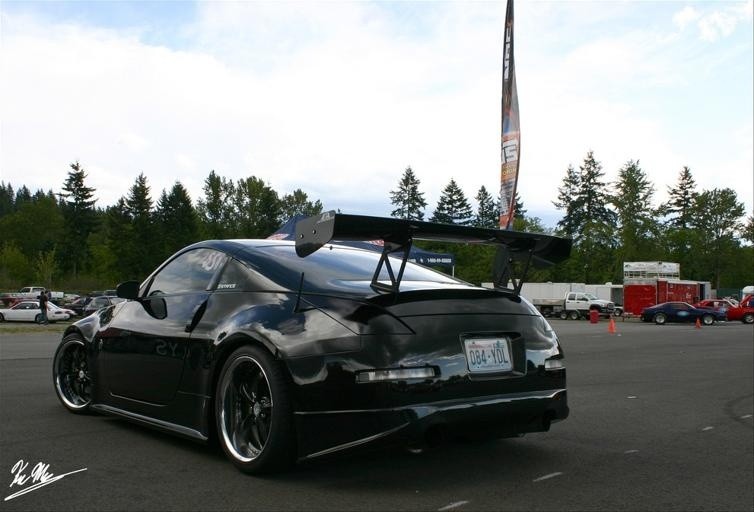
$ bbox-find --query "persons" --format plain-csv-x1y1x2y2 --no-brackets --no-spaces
83,293,91,305
47,290,51,300
36,291,47,325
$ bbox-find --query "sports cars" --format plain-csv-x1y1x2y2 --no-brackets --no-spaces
52,208,573,478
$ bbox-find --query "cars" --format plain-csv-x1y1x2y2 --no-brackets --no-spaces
0,286,125,315
0,301,70,325
640,301,727,327
691,296,754,325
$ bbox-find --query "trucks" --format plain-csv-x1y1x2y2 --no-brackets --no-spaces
533,293,615,322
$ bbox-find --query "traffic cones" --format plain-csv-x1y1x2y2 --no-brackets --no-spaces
607,318,616,336
695,318,702,330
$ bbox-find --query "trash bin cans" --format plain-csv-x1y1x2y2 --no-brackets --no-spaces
590,309,598,323
